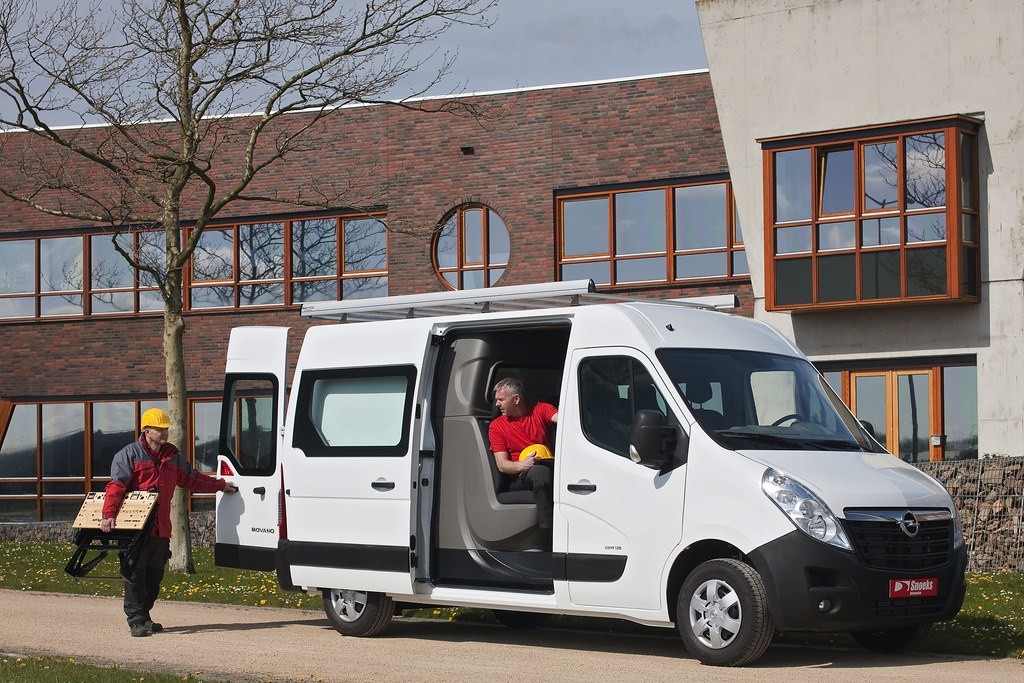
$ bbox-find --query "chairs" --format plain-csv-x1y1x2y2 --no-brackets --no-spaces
684,370,729,431
627,373,665,426
481,397,538,505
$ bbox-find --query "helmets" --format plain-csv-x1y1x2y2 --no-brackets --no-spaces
518,445,555,477
140,408,173,429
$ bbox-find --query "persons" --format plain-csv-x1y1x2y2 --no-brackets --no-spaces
488,377,559,552
100,407,236,637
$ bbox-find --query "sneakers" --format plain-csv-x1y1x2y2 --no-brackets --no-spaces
131,624,148,637
141,618,162,631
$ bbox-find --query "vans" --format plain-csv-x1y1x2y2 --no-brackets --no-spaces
214,281,969,666
